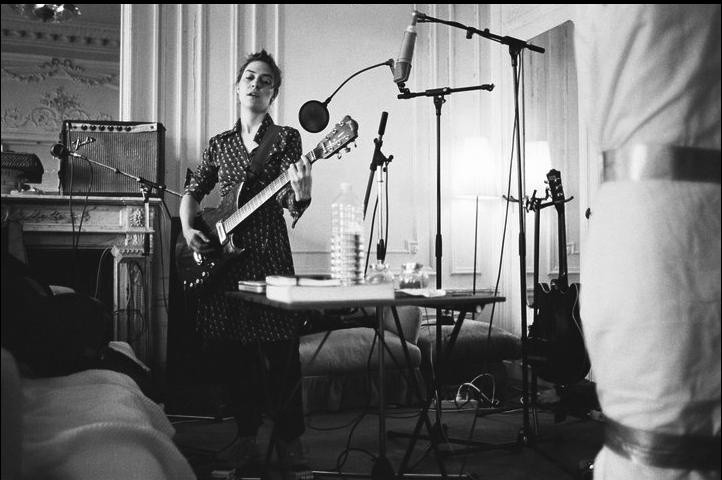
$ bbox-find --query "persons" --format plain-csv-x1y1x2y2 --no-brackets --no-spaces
179,49,312,465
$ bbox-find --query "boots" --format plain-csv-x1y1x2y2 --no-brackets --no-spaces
210,434,256,480
275,436,314,480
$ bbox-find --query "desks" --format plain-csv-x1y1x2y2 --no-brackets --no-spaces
224,288,506,479
1,188,171,373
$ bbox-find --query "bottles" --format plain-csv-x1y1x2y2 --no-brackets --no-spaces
398,263,429,290
329,183,364,285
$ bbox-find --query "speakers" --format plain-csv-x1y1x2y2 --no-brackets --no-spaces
61,114,168,194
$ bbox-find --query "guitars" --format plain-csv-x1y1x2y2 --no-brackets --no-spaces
528,169,590,384
175,116,358,295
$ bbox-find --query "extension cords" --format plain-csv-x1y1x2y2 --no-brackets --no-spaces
435,399,478,410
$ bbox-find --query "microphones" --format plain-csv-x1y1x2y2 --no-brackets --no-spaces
50,142,75,158
393,9,422,82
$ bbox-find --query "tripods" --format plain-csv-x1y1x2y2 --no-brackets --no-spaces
391,83,503,478
430,13,597,477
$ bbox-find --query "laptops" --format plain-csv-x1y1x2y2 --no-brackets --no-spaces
238,281,267,295
